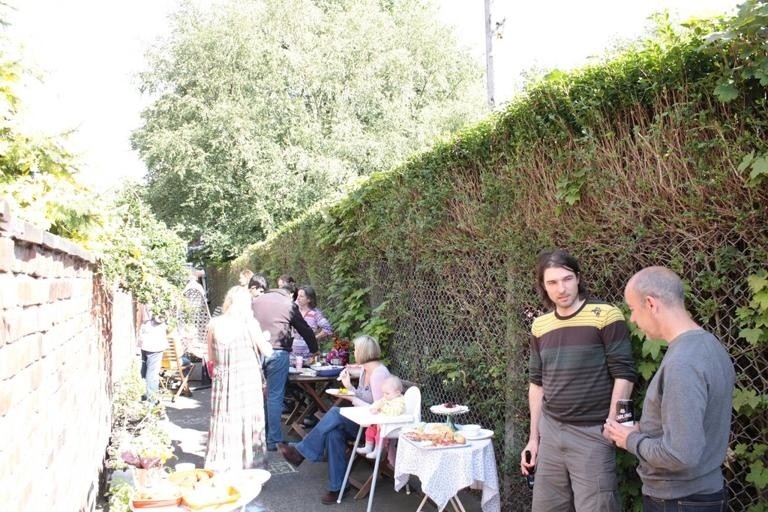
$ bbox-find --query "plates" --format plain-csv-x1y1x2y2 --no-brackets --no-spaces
325,389,355,396
456,428,495,439
131,469,271,510
286,367,301,374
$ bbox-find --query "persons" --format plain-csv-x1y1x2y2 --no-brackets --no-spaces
139,301,170,405
276,335,392,504
356,375,405,459
520,250,638,511
604,265,735,512
204,269,332,472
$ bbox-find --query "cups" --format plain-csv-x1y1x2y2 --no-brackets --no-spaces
464,424,482,437
174,462,197,471
295,356,303,371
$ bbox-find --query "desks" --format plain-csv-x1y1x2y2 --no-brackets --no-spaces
282,349,504,512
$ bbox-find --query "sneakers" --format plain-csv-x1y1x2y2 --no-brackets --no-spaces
275,443,303,467
322,487,350,505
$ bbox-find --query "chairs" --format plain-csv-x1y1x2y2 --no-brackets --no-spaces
156,332,197,405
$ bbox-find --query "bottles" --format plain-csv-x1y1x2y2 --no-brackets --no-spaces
525,450,536,490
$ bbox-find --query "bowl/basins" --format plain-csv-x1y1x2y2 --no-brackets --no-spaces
314,366,345,376
